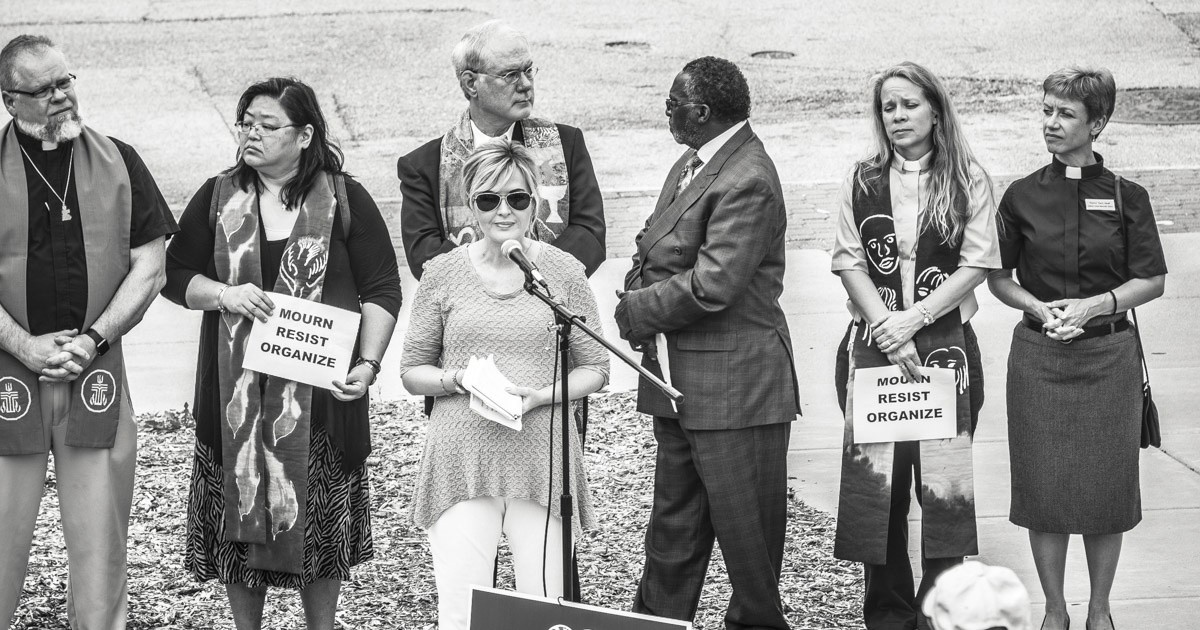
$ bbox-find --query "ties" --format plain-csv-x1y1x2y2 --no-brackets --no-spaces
673,153,700,201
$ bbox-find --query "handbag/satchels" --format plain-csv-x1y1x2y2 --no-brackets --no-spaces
1140,383,1162,449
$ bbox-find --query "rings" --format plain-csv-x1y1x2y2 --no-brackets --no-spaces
899,363,907,371
887,340,891,346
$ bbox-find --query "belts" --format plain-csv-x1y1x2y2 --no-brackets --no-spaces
1021,316,1129,345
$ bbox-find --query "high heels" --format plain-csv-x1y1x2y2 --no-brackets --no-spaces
1086,611,1116,630
1040,611,1071,630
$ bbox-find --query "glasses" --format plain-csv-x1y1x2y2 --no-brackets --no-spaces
3,71,77,100
473,191,534,213
666,98,707,111
235,121,299,137
472,65,539,86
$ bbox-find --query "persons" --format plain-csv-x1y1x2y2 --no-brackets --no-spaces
160,79,403,630
988,65,1169,630
0,34,180,630
614,58,802,630
401,141,608,630
831,62,1003,630
396,22,607,282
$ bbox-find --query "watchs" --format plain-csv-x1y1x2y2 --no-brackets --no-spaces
453,368,471,395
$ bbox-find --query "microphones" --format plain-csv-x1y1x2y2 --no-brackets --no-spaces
500,239,548,288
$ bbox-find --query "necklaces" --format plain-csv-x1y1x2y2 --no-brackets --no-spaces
20,144,74,221
267,189,287,210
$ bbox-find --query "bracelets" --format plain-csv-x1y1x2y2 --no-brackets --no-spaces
217,285,230,314
1107,290,1118,317
913,301,934,326
440,370,453,397
84,328,110,357
356,358,381,385
